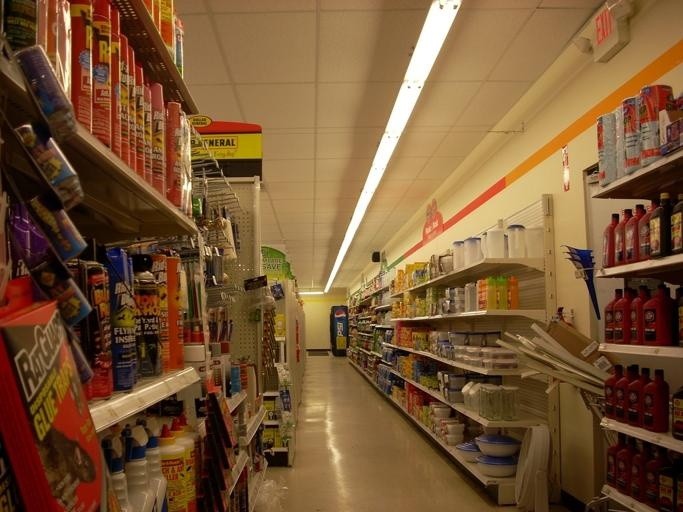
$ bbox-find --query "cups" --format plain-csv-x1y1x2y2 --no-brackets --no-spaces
450,224,544,269
435,330,499,360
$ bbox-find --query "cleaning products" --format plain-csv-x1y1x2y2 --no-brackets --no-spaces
102,412,202,512
602,192,683,510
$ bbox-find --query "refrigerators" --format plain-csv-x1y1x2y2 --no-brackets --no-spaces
330,303,348,357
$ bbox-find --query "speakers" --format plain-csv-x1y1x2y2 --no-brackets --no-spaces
371,252,380,262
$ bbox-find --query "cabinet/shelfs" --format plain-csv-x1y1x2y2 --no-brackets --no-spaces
0,0,306,512
582,146,683,512
347,194,562,505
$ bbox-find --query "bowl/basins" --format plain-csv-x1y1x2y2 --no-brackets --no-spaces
456,425,521,476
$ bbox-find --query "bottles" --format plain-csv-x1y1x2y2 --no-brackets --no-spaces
649,188,682,512
453,275,518,312
240,364,249,389
11,0,212,400
159,409,203,512
230,361,241,393
372,327,403,396
458,380,519,421
